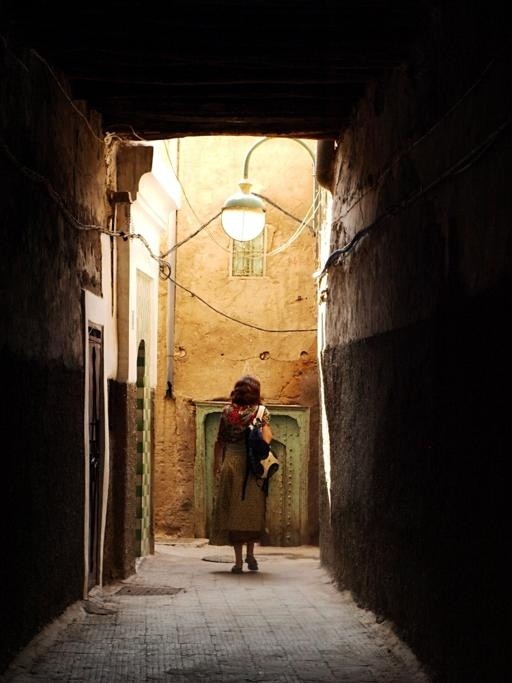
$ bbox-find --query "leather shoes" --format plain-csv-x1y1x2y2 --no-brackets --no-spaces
231,560,243,573
245,556,258,570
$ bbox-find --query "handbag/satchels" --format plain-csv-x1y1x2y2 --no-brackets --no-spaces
243,428,279,480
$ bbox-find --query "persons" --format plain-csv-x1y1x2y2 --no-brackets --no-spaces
208,375,273,573
